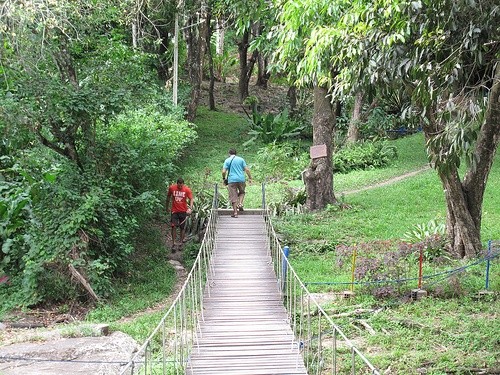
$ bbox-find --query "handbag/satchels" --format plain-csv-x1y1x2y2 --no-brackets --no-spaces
224,179,228,185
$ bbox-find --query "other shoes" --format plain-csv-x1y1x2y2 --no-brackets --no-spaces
231,213,238,217
172,244,177,250
238,203,243,211
179,243,184,250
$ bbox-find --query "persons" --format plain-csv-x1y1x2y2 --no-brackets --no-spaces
165,178,193,252
222,149,253,217
